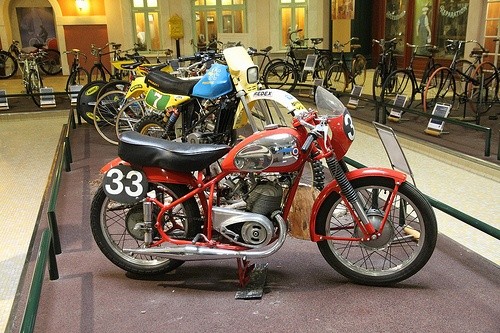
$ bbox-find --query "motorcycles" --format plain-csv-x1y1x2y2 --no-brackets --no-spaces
90,86,437,286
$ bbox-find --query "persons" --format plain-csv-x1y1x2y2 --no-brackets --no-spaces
198,35,205,53
208,34,218,52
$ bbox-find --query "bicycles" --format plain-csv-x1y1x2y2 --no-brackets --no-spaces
62,49,90,101
89,41,139,81
0,40,62,79
17,46,43,107
77,29,499,191
